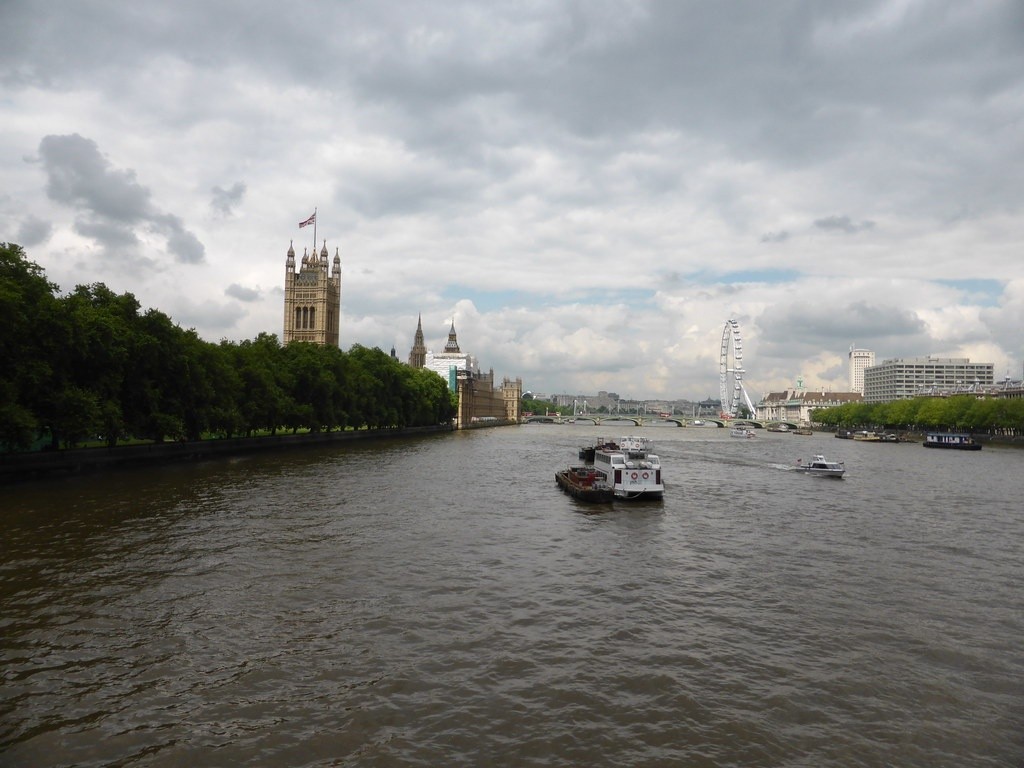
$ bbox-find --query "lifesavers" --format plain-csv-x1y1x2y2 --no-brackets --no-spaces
642,472,649,479
631,473,638,479
621,443,625,447
635,444,639,448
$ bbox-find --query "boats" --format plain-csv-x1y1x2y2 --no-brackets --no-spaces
922,432,983,451
554,435,666,503
854,430,880,441
794,454,846,478
835,429,854,438
685,419,706,426
578,436,620,464
767,426,791,432
793,429,813,435
729,427,757,438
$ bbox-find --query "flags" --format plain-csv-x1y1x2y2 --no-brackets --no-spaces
299,213,315,228
